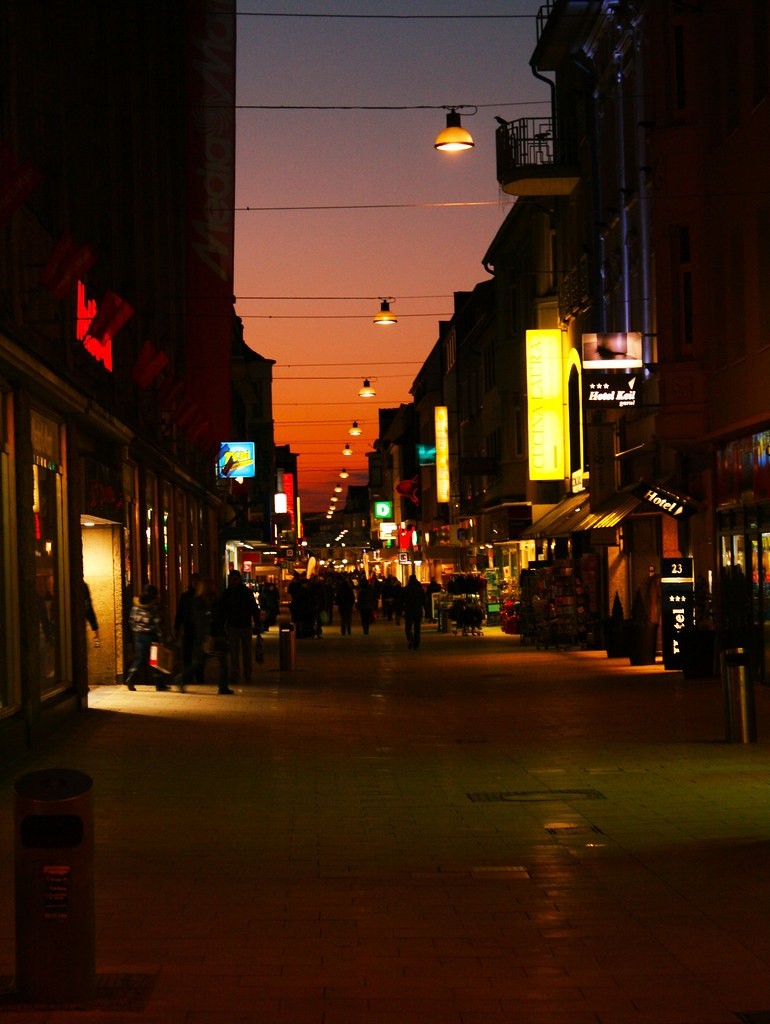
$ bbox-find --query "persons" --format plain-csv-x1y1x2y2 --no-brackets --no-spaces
288,572,403,639
258,582,281,628
402,574,431,649
84,583,100,643
126,584,172,691
449,575,482,636
173,569,261,695
426,577,441,593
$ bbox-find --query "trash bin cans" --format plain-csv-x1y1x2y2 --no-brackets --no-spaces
14,769,95,998
720,647,757,743
279,622,295,670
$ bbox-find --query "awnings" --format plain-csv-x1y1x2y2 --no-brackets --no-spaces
521,493,590,539
570,476,672,531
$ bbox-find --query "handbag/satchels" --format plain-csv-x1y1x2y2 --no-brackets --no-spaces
254,635,264,665
149,641,175,674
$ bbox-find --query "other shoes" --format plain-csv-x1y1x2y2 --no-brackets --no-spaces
217,686,234,695
175,674,185,694
125,678,136,691
156,683,172,690
